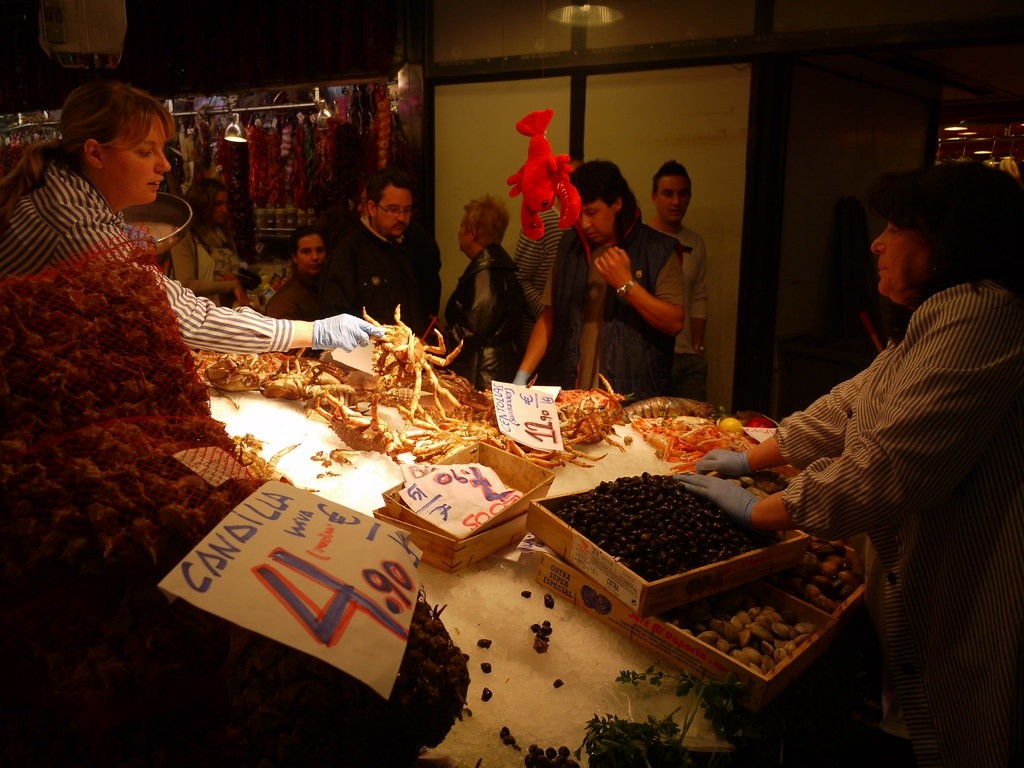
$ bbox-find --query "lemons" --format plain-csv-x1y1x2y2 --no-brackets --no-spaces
720,417,745,434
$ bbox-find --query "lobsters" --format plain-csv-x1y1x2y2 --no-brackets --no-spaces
507,109,581,240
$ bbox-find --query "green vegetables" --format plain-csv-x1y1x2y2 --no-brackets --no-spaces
575,665,746,768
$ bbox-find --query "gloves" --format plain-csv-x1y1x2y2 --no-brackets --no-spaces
312,314,384,352
673,475,758,529
695,449,750,476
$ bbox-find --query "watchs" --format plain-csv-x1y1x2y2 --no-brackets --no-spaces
616,279,635,298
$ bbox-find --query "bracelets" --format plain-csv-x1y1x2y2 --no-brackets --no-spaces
695,345,704,351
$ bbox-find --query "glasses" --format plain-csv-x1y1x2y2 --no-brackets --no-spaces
374,202,416,217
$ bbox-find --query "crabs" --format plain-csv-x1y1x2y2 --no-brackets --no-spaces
181,304,762,476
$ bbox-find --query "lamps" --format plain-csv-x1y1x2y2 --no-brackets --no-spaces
317,108,339,131
224,113,248,142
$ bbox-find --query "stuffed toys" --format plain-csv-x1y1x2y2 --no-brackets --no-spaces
507,108,581,241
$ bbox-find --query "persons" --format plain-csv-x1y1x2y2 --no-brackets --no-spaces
511,159,685,403
514,192,574,386
331,167,441,342
0,80,384,355
265,224,338,322
169,178,248,309
673,158,1024,768
445,196,520,392
641,160,709,402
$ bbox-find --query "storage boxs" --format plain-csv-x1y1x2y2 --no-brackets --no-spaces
706,470,866,625
373,506,532,575
525,471,810,617
533,544,839,711
381,441,556,542
